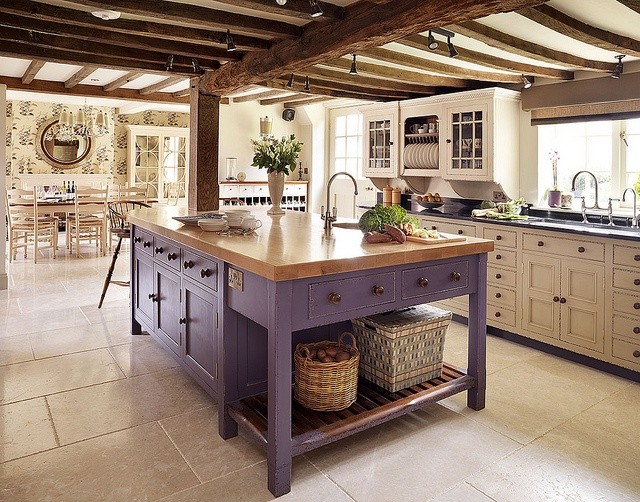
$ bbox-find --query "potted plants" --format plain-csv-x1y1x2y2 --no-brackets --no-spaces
544,151,564,207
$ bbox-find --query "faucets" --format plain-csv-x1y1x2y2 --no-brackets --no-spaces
571,171,599,209
622,188,637,226
320,172,358,235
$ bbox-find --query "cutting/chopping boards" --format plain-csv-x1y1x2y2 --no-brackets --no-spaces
407,232,467,244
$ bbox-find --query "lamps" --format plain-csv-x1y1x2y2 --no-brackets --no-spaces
428,30,439,49
165,55,174,68
287,74,295,88
192,58,201,72
58,98,110,138
45,126,76,142
226,29,235,52
522,74,531,89
611,55,625,79
304,76,310,89
350,56,357,75
309,0,323,17
447,36,458,57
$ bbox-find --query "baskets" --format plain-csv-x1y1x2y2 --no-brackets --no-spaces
351,303,453,394
293,331,360,412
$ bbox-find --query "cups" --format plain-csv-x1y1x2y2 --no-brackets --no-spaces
475,138,481,149
418,126,426,133
463,139,472,149
410,124,420,133
429,123,437,133
561,194,573,209
421,124,428,133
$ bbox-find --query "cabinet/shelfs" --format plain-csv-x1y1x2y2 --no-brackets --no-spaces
182,244,268,401
219,181,307,212
445,87,522,180
605,238,640,373
476,222,518,335
19,174,113,235
152,236,181,368
130,223,153,336
293,253,477,331
419,216,476,319
518,226,605,362
360,101,398,179
398,95,444,177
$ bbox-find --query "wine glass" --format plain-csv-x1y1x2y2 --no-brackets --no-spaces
51,183,58,199
43,185,49,199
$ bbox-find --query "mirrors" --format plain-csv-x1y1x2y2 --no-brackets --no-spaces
35,116,95,168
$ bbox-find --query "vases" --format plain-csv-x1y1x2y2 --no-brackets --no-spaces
266,172,286,214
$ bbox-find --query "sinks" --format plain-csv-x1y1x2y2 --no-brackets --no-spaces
331,222,358,231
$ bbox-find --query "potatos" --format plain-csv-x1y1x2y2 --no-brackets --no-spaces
366,233,393,243
385,224,407,244
302,346,351,364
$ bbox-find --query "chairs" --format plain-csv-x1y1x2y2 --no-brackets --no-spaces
24,212,59,250
218,198,244,206
98,201,152,309
153,182,181,206
109,184,149,251
69,185,109,258
6,186,56,264
66,212,99,249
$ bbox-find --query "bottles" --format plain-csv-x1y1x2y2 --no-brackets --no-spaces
66,181,71,200
71,180,75,198
298,161,303,181
392,188,401,204
383,184,392,202
62,181,66,200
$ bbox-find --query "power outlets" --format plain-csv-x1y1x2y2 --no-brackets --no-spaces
228,269,243,291
493,190,506,202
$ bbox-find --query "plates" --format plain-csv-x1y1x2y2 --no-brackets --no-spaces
227,225,242,228
416,200,444,209
223,210,253,230
403,143,439,169
198,219,228,232
172,214,223,226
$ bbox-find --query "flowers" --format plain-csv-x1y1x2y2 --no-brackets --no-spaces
250,135,303,176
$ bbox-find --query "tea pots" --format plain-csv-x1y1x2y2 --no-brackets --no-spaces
239,215,263,232
